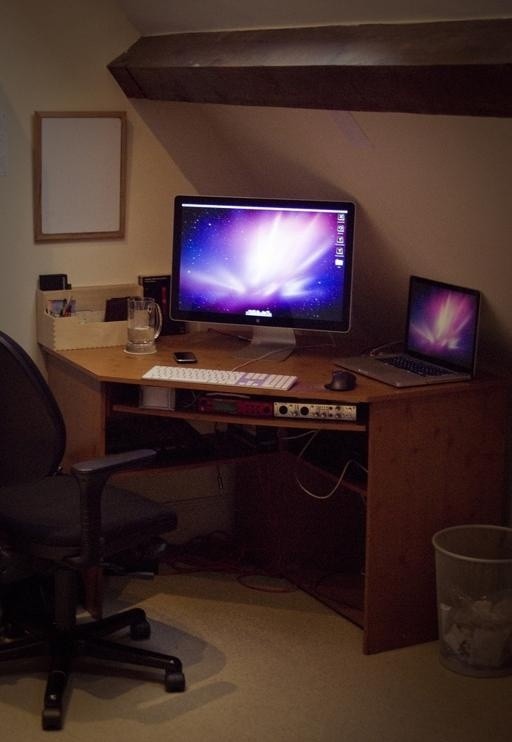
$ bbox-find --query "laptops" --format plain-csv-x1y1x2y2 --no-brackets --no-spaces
334,274,482,388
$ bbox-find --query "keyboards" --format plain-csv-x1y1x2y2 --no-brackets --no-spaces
140,365,299,392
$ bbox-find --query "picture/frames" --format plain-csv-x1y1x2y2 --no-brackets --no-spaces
34,111,128,244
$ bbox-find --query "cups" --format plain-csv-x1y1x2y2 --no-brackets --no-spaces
123,296,162,355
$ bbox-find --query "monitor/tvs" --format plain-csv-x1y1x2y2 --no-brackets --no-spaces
167,194,356,360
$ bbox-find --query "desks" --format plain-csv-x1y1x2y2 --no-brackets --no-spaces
42,332,512,655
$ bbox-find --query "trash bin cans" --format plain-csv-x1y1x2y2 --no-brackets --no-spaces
432,525,512,680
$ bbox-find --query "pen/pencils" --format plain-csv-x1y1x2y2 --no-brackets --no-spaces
60,295,73,316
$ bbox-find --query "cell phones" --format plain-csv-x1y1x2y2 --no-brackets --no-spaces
173,351,197,364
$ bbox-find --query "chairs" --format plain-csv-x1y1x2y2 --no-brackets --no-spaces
0,330,183,730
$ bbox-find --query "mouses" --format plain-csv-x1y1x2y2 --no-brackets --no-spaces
324,370,357,392
